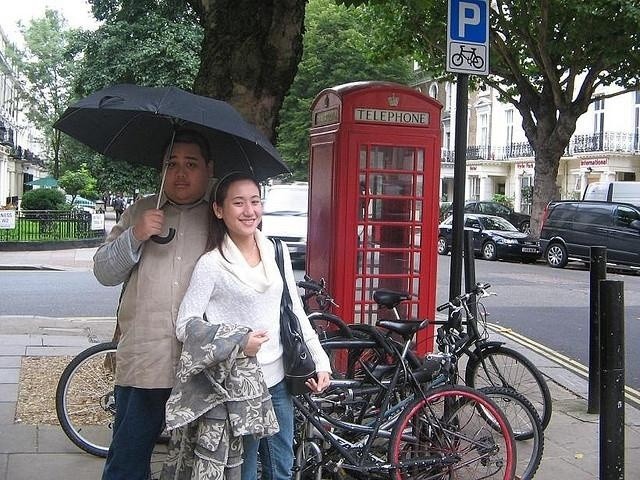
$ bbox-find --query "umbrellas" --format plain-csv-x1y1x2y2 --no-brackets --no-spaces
23,175,59,188
50,85,291,245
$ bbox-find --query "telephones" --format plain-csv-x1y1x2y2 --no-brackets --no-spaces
359,179,370,208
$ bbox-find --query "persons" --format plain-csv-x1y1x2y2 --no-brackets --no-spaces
174,169,333,480
92,128,227,480
114,196,124,224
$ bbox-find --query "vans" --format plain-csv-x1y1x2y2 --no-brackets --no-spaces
540,181,640,268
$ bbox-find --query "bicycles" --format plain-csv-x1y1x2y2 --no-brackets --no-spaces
372,280,553,442
56,274,546,480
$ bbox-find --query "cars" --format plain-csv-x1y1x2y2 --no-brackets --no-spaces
260,182,308,269
437,201,542,263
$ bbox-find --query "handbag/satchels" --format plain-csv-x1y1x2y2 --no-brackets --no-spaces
267,237,318,395
